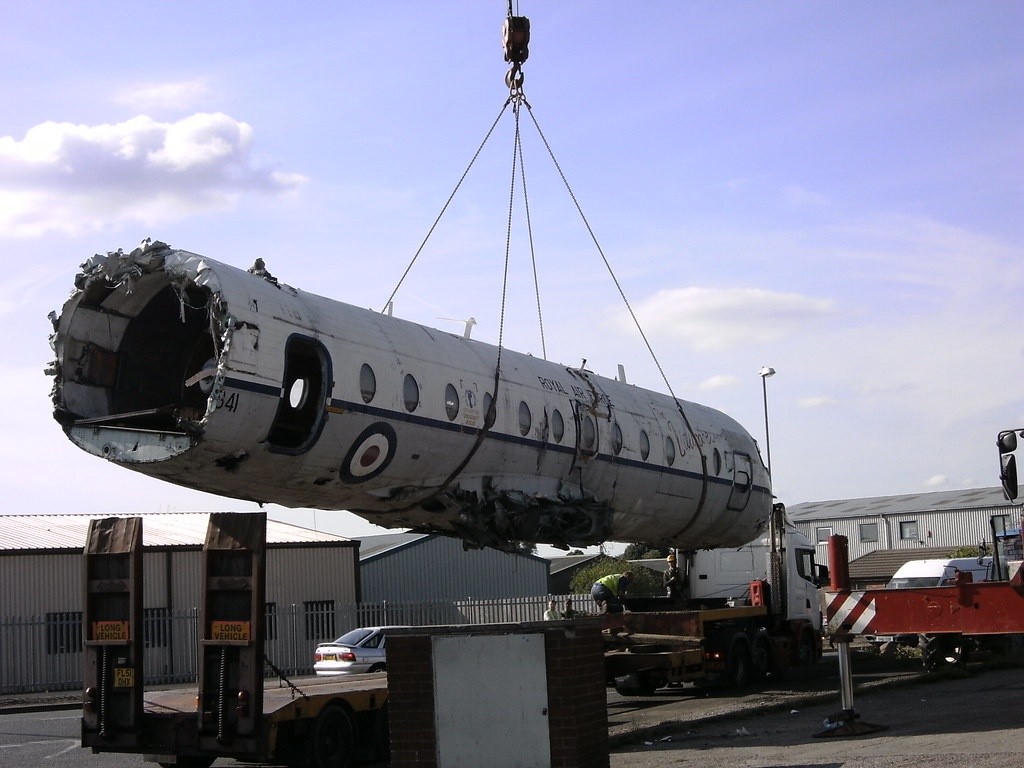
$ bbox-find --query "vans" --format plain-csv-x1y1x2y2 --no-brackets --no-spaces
885,556,1005,586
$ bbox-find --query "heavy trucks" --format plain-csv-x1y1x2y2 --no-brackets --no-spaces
81,502,828,768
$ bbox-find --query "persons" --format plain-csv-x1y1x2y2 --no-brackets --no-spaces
591,572,634,617
561,599,578,618
544,600,562,621
663,554,686,606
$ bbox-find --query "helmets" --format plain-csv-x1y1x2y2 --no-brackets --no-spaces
666,555,675,562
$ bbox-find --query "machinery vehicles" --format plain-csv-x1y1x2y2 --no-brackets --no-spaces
811,427,1024,740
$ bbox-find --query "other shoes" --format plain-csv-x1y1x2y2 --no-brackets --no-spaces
602,600,608,613
597,600,602,613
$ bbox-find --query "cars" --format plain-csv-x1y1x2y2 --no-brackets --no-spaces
313,626,412,676
